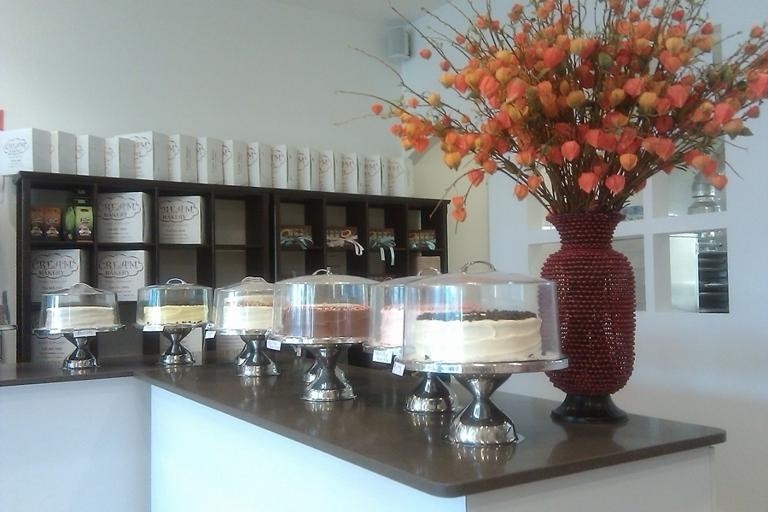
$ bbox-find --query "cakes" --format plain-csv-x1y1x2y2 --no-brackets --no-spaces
280,303,371,338
45,306,115,330
380,303,477,347
223,294,275,330
143,305,206,324
414,309,542,363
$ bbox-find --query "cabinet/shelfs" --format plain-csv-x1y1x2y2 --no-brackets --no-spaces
5,170,270,383
528,23,729,313
271,188,451,384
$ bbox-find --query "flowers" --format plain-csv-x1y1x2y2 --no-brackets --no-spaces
332,0,768,234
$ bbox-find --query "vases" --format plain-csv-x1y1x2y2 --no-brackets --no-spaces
537,210,636,424
